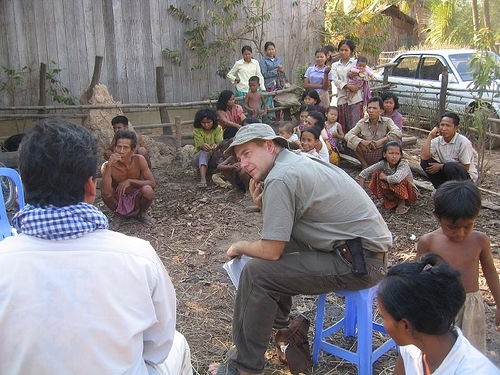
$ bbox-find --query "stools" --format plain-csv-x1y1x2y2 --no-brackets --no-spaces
311,277,401,375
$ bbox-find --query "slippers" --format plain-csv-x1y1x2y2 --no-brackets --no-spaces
395,206,410,214
135,214,156,227
109,215,126,231
198,182,208,188
208,362,240,375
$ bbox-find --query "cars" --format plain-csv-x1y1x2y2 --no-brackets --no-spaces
370,49,500,149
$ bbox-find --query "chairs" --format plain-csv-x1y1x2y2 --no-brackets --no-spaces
424,65,442,80
0,168,25,241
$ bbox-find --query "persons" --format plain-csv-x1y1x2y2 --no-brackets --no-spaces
227,45,266,112
420,113,478,189
209,123,392,375
380,94,402,131
104,116,151,168
245,76,266,123
304,39,368,131
278,89,344,166
193,109,259,192
377,253,500,375
217,90,246,138
101,130,155,231
357,142,418,214
259,42,282,121
344,96,402,168
416,180,500,356
0,120,193,375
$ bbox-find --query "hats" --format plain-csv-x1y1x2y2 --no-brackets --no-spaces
223,123,288,159
